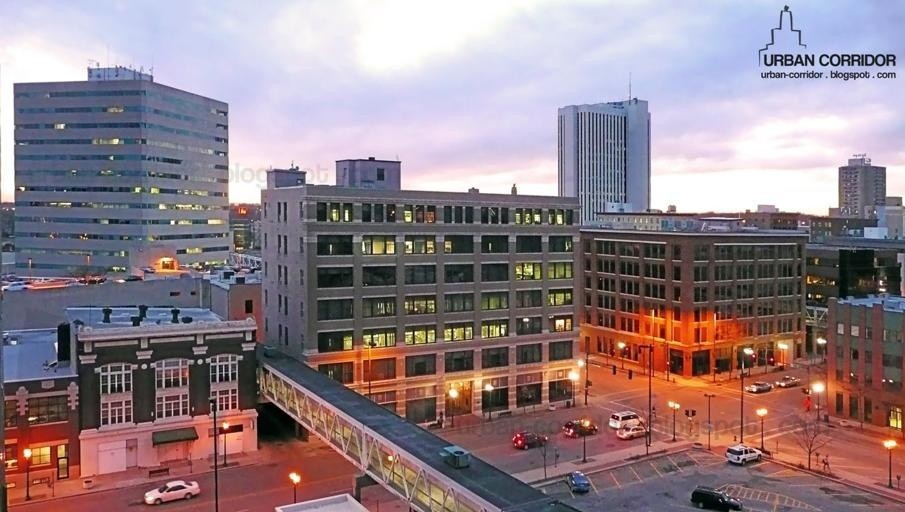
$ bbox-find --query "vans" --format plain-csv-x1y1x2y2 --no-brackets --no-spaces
608,411,642,428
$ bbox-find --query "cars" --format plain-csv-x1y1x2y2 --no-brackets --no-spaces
617,426,646,439
775,375,800,388
2,281,30,290
746,382,770,393
567,470,589,493
563,420,597,438
512,431,547,449
144,480,200,505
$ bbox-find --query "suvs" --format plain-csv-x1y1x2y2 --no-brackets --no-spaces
692,486,741,512
726,445,762,465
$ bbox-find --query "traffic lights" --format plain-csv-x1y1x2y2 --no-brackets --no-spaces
685,408,697,416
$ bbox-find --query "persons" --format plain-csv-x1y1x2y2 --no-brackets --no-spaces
821,452,832,474
803,394,813,416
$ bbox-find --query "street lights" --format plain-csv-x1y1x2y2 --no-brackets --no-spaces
486,385,495,420
24,449,31,500
223,422,228,464
638,344,654,447
582,420,590,461
569,372,578,406
740,372,748,443
813,383,825,419
369,341,376,400
668,401,679,441
757,409,768,449
818,339,827,364
289,473,299,504
703,394,715,449
776,343,787,367
619,343,625,369
745,349,754,376
450,388,457,427
885,440,895,488
